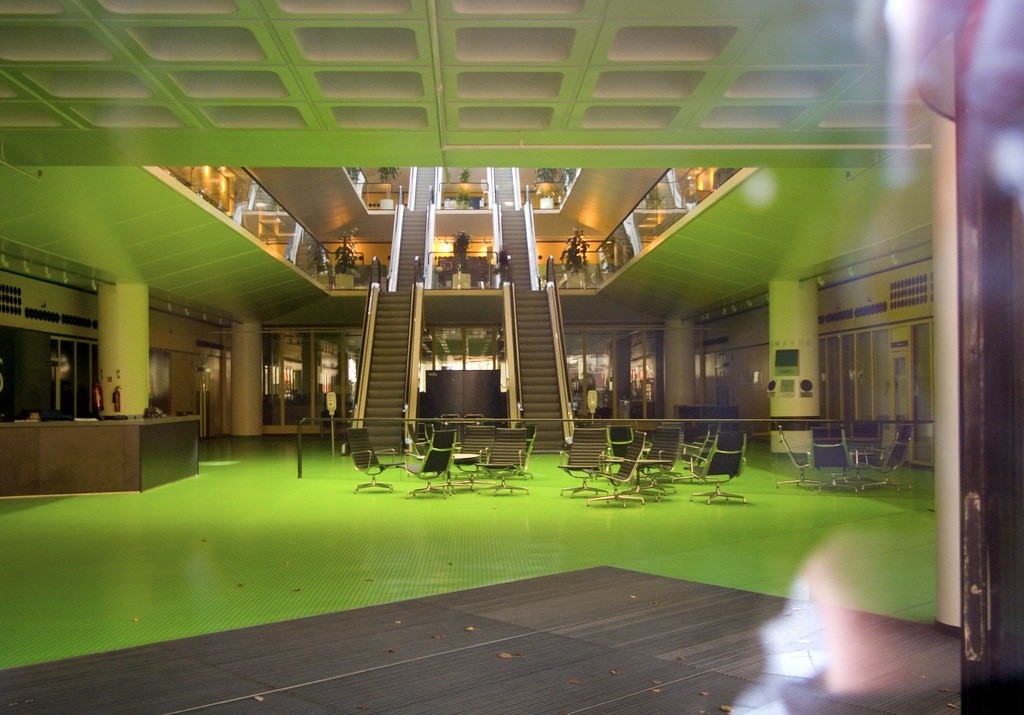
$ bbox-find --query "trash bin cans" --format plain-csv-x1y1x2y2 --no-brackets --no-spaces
618,400,631,419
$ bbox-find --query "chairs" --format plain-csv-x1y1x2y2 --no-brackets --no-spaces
775,422,914,495
343,413,540,500
557,425,748,508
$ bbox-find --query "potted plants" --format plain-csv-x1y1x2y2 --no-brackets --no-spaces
450,231,472,290
532,168,560,209
377,167,401,209
334,227,359,289
560,225,590,288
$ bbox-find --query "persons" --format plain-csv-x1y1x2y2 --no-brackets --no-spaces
498,245,512,288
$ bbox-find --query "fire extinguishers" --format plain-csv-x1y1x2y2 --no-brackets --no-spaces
112,385,122,412
92,378,104,411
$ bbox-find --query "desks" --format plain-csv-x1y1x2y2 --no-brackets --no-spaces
813,438,882,490
636,460,673,503
451,454,481,494
